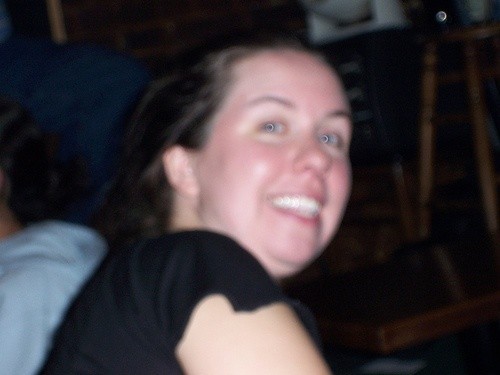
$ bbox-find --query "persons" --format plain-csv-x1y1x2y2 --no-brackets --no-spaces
1,96,111,375
30,25,357,375
